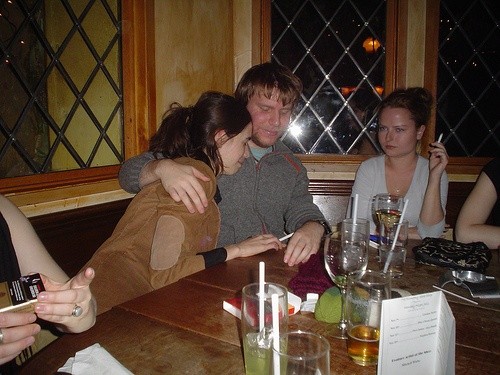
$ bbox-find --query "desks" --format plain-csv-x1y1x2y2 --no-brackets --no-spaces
15,239,500,375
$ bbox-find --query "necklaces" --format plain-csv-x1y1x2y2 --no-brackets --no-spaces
395,187,400,192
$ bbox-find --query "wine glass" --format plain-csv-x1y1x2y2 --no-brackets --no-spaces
323,230,369,340
373,193,404,246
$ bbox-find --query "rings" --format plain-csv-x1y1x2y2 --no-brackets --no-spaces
0,328,4,344
72,304,82,317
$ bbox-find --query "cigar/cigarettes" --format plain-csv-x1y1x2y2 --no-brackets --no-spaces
438,132,443,143
278,231,295,241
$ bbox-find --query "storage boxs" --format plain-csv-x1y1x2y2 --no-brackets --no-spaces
223,284,302,326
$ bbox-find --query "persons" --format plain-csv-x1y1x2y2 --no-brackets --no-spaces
77,90,286,316
118,62,332,268
0,194,97,375
345,87,449,240
455,157,500,250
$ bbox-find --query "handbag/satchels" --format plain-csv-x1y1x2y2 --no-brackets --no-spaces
411,236,492,273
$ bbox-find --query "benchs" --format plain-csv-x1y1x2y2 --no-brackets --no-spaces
27,180,500,331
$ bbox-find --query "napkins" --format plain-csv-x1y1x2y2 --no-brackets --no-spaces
57,343,133,375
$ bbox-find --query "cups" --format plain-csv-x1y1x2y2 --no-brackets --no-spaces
341,218,370,255
272,330,331,375
344,269,392,367
240,282,288,375
379,218,409,278
